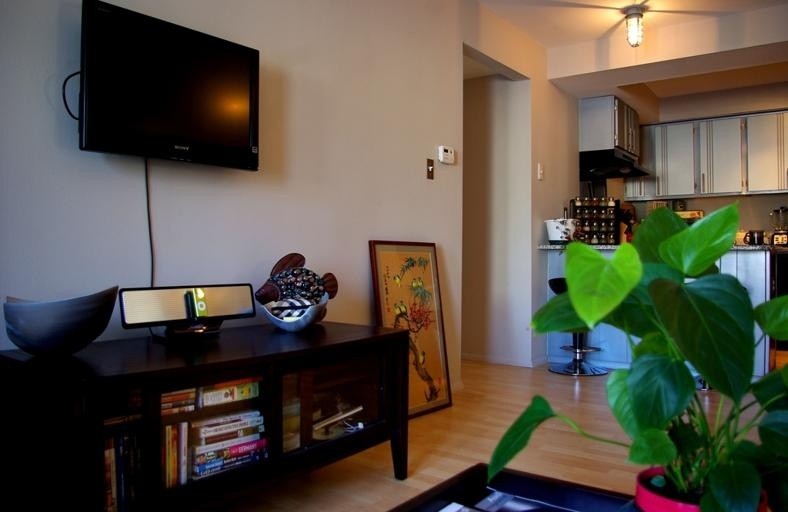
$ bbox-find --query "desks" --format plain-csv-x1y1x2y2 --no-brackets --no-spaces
383,458,639,511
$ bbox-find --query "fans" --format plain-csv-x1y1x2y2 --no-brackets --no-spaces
525,0,735,44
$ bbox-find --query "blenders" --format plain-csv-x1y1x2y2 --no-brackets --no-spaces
768,206,788,247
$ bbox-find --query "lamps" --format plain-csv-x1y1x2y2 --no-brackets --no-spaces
624,11,648,49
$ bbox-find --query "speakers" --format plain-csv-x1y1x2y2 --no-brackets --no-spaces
119,283,256,337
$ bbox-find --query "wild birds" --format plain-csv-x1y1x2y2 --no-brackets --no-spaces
410,275,425,291
393,274,403,288
392,299,410,320
418,349,426,365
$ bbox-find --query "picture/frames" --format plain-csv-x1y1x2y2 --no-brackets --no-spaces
367,238,454,419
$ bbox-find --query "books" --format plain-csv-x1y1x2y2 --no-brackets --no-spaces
86,369,270,511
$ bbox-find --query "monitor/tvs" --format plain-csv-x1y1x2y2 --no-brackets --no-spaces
77,0,260,172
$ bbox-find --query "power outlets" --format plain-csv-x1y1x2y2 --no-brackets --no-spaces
537,164,545,180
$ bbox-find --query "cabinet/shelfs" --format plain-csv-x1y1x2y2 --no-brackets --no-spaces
622,108,788,203
0,320,411,512
579,93,641,163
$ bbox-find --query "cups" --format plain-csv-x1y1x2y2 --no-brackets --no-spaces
743,229,764,245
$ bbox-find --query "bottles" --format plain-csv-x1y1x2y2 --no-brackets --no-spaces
574,196,616,245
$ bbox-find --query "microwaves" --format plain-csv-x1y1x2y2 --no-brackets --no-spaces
674,210,704,227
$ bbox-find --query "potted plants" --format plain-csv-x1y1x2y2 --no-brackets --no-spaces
483,209,787,511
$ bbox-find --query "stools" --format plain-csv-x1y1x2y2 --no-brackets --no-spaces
548,275,608,376
